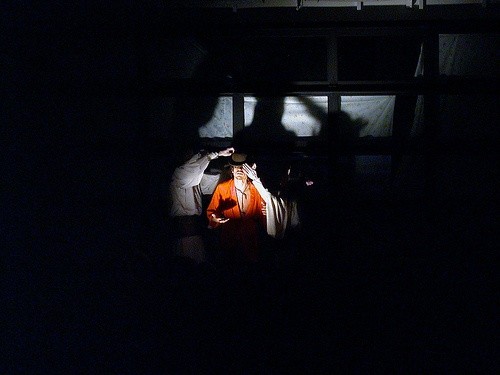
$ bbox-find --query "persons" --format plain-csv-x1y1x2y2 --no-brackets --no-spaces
243,160,303,264
207,147,270,260
171,143,235,265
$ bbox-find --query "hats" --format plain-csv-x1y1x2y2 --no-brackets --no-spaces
226,153,253,168
